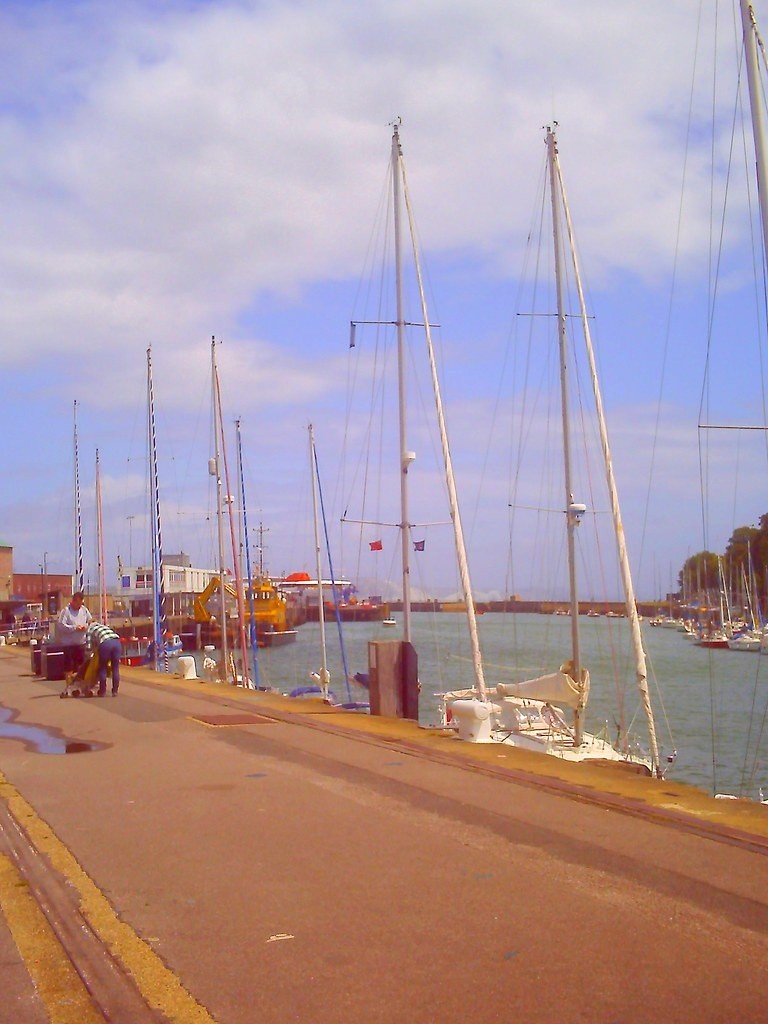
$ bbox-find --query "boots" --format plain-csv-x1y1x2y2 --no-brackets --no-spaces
63,671,74,687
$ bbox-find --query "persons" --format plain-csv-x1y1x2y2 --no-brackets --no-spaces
22,612,31,635
9,613,15,634
356,596,370,605
57,591,94,696
86,618,122,698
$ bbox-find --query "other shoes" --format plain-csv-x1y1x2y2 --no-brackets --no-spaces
97,690,104,697
111,689,117,697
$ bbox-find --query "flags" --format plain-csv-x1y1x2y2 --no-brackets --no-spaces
413,540,425,551
226,568,232,575
369,540,382,551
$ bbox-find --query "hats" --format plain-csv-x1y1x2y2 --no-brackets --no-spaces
88,618,97,627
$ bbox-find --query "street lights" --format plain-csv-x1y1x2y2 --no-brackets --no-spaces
126,515,136,567
38,563,44,620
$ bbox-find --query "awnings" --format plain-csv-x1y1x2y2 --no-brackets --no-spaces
0,601,28,610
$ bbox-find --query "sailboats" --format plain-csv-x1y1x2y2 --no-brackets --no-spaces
66,332,370,718
554,531,768,655
328,114,677,785
671,0,768,806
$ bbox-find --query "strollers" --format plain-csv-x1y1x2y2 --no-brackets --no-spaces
59,644,112,698
